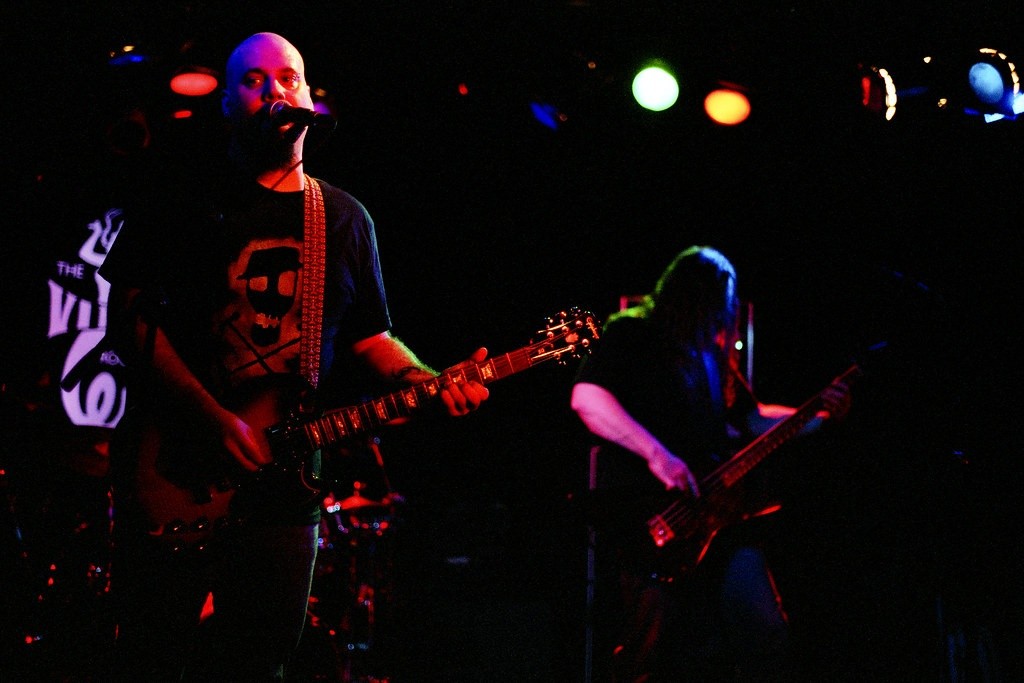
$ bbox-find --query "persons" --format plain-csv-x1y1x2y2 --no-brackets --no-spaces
570,247,852,683
133,32,491,683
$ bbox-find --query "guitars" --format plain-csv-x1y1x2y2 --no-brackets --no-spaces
620,365,872,591
140,305,604,537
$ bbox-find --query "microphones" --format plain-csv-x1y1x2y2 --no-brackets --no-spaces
269,100,338,130
